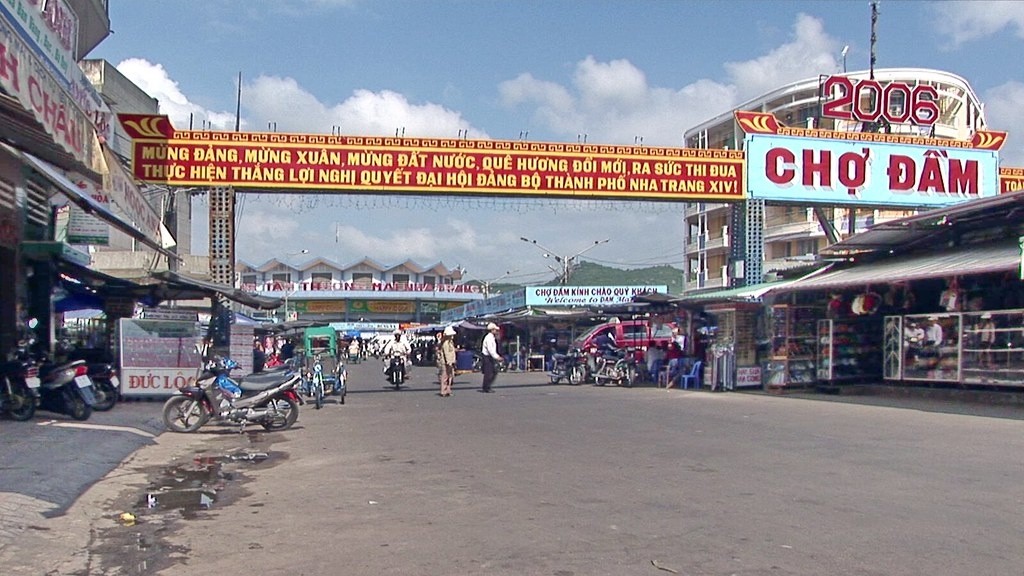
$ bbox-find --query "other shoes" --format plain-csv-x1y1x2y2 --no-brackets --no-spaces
483,389,495,393
404,375,410,380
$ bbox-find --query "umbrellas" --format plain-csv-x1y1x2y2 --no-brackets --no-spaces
590,301,675,355
269,319,329,347
631,292,679,305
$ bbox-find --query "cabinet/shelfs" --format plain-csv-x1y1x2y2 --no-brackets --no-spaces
706,303,1024,388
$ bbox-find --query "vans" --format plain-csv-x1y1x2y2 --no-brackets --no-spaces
566,316,681,361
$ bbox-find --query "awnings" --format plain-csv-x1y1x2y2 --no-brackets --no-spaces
737,242,1024,299
151,270,285,313
667,271,814,307
409,319,487,337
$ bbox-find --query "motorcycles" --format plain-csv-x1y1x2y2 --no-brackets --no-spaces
384,350,411,391
162,324,350,437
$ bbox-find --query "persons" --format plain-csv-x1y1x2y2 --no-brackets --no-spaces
979,311,996,369
436,326,456,397
383,329,412,366
348,335,389,360
646,340,687,379
481,321,504,393
252,335,296,374
903,316,944,371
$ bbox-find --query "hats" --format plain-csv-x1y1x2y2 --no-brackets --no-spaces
394,329,402,336
928,318,934,320
910,323,917,327
445,326,457,336
980,313,992,319
909,338,918,343
932,317,938,321
487,322,500,330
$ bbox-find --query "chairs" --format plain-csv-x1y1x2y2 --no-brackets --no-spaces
637,357,701,389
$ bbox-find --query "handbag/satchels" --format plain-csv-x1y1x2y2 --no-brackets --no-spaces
445,364,452,375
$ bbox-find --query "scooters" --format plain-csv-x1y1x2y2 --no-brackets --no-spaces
0,316,120,422
547,342,637,388
